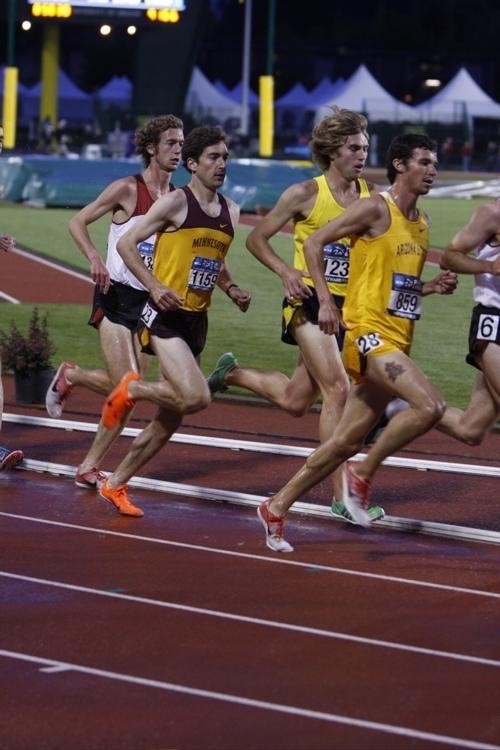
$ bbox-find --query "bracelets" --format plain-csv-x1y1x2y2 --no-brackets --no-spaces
226,283,238,297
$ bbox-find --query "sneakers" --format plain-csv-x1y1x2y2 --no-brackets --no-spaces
343,459,374,529
203,353,241,399
98,477,144,518
257,494,295,557
331,495,386,525
43,360,78,419
362,399,399,448
76,465,107,490
1,447,25,474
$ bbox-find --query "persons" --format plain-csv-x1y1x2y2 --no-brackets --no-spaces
365,196,499,446
205,105,388,523
0,125,25,472
97,124,251,517
258,134,459,552
49,117,500,176
46,114,187,490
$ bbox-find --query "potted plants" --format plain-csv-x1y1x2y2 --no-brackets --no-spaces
0,303,59,406
